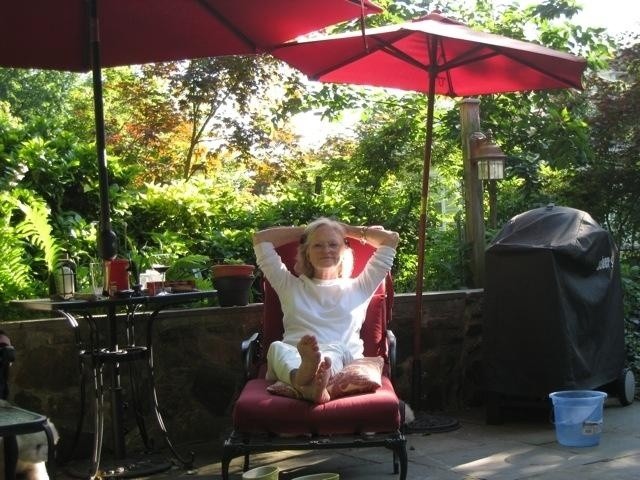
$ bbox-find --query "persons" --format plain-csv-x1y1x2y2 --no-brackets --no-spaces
251,217,401,404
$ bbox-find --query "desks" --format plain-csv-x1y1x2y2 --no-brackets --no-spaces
5,288,215,480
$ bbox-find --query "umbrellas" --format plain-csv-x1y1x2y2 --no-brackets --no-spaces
270,8,591,403
1,0,386,264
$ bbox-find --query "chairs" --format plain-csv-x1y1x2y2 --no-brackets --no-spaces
221,228,410,480
0,332,57,480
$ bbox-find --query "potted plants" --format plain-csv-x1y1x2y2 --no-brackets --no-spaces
213,255,256,308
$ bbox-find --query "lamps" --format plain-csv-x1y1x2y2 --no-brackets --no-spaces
469,127,506,182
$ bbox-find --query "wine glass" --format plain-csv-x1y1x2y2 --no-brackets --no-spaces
88,263,107,300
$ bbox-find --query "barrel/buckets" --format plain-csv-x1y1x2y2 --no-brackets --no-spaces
547,390,608,447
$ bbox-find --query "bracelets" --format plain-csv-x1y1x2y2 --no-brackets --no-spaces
360,225,370,246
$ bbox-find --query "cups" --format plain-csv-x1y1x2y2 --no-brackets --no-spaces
149,250,173,297
